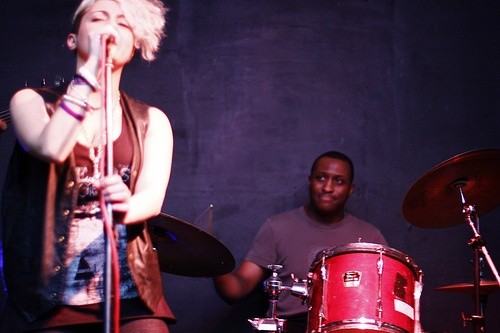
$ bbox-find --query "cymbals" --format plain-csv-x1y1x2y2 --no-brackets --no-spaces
402,147,500,228
435,281,500,293
146,213,235,278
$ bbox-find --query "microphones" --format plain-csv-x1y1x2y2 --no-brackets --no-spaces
101,33,114,45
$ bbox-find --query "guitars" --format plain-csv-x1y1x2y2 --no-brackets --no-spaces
0,76,67,122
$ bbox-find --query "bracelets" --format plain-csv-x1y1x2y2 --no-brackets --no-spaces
60,64,102,125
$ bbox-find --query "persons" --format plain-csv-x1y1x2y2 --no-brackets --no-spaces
212,150,387,333
0,1,179,333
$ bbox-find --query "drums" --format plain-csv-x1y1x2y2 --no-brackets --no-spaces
307,242,422,333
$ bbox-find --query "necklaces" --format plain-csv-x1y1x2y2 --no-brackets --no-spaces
82,88,122,179
